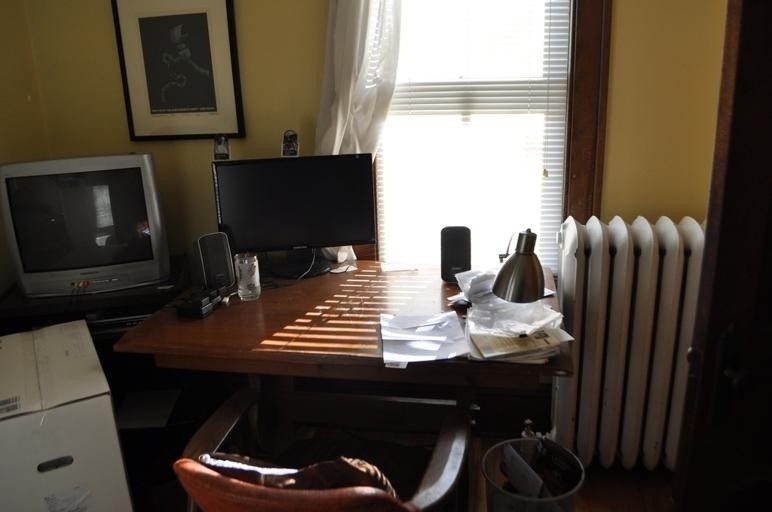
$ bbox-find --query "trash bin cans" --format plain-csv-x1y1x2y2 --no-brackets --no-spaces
482,437,585,512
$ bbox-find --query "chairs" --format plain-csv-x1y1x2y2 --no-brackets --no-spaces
171,378,486,512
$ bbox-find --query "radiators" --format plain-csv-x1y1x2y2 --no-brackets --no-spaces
553,214,708,479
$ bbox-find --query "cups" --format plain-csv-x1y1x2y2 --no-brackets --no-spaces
234,253,261,302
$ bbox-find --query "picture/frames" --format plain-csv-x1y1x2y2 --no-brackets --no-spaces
113,0,245,143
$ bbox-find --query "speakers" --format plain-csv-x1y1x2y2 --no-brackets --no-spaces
441,226,471,283
191,231,236,298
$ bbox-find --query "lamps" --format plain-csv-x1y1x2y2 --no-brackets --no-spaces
493,230,545,306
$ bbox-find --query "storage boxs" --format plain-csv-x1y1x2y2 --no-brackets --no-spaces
2,320,136,511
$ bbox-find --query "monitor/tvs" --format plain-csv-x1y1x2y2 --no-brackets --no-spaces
212,153,378,279
0,153,173,299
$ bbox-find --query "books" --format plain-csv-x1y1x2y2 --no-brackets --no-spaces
504,441,545,499
381,267,575,369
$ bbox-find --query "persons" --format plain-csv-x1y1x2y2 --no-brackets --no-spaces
159,25,210,106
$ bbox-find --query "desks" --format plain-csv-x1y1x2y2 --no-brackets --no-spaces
112,261,577,510
